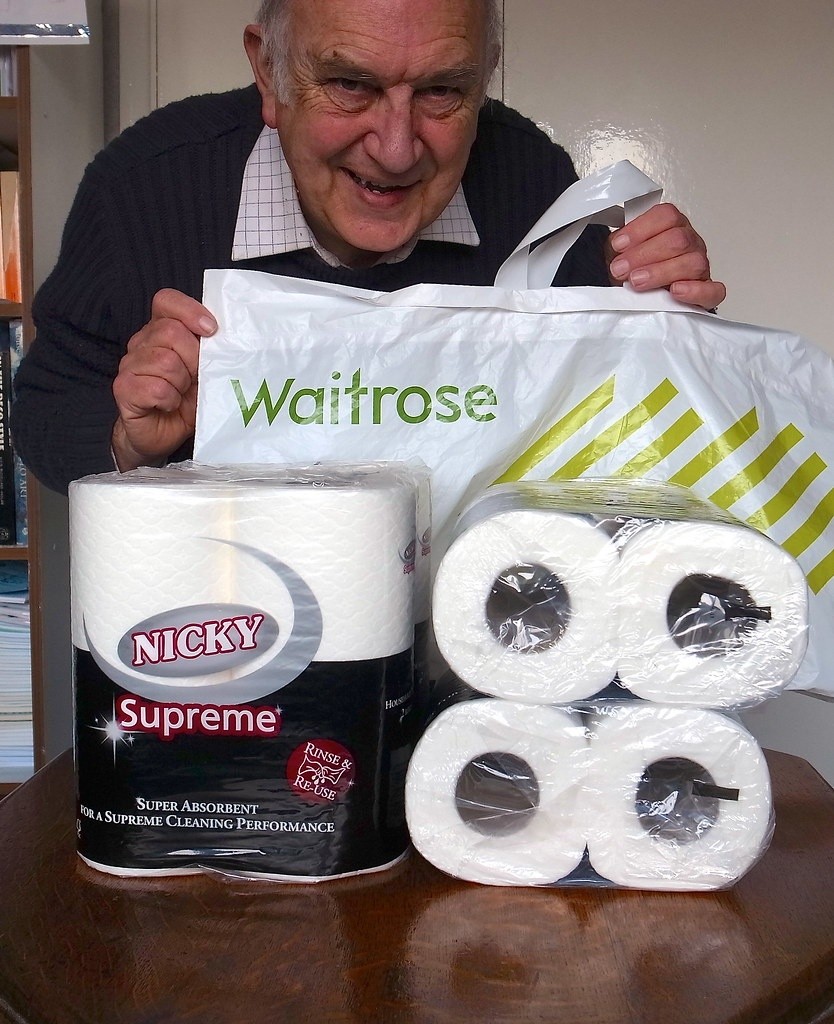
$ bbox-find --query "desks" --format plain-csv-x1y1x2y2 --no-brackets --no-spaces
0,748,833,1024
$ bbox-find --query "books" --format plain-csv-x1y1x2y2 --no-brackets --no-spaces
1,171,22,304
0,317,28,545
0,561,34,783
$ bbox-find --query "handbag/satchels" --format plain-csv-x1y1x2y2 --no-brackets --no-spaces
191,157,833,699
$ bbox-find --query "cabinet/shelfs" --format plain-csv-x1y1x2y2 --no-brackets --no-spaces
0,43,45,803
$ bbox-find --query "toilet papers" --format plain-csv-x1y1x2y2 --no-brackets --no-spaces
67,460,811,892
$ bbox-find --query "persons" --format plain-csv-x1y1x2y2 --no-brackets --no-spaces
10,1,728,496
